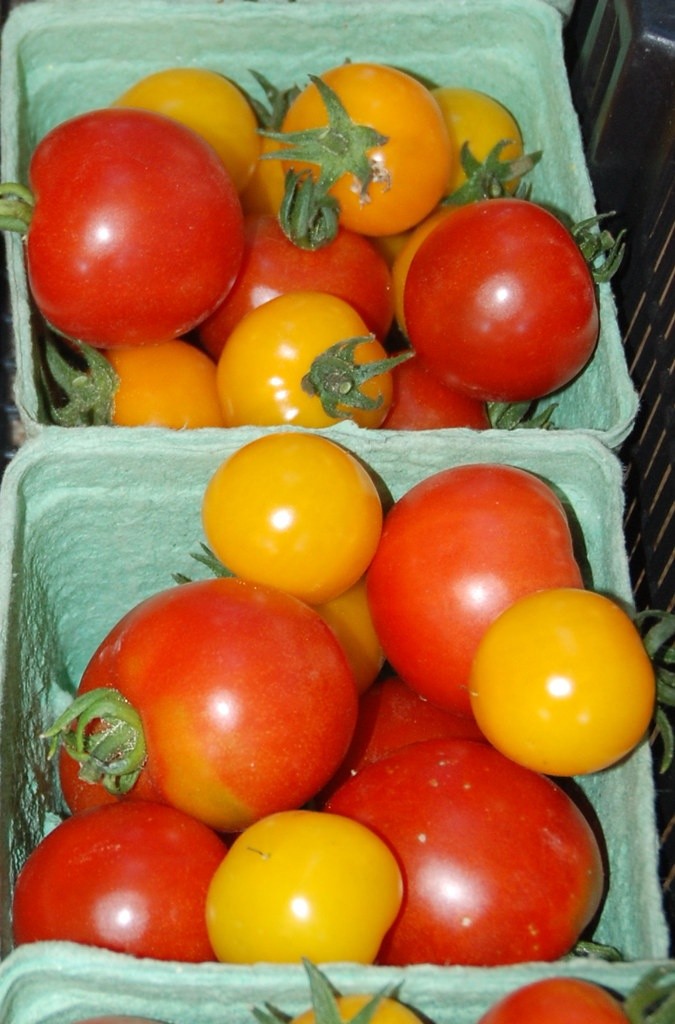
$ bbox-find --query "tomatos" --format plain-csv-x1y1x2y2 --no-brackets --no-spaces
12,433,657,1023
25,61,600,431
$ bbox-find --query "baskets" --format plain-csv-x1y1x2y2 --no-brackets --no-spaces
0,0,641,452
0,429,671,965
0,938,673,1023
